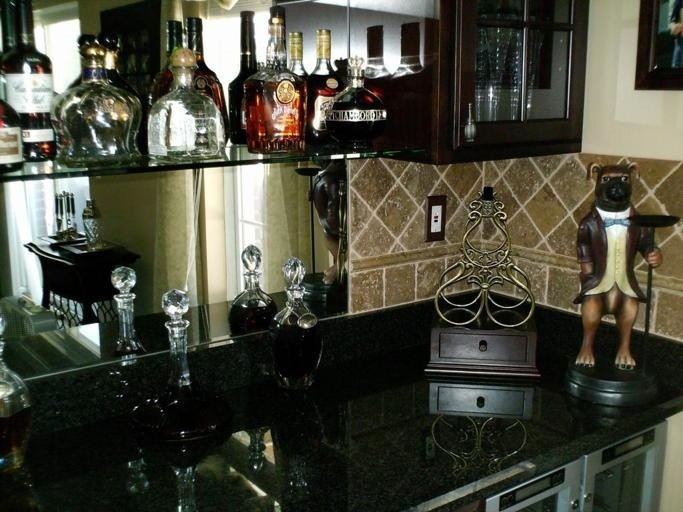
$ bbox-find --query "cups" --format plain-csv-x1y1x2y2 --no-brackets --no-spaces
475,13,542,121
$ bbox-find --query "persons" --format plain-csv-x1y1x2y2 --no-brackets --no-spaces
668,0,683,69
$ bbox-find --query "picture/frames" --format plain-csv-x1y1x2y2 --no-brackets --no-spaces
632,0,682,92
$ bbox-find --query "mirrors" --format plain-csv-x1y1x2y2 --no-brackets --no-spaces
1,0,350,382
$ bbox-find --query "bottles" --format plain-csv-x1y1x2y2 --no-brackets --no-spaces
363,23,393,83
327,54,388,150
244,4,307,153
305,28,342,149
287,31,308,76
461,103,477,144
129,288,234,444
228,10,261,144
125,400,325,512
391,20,428,146
228,245,278,337
0,312,36,474
106,266,147,356
264,257,322,397
81,198,105,251
0,0,228,174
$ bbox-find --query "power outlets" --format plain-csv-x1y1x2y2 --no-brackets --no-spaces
425,193,446,241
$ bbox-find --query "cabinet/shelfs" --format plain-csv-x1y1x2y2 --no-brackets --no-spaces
350,0,589,167
1,284,681,512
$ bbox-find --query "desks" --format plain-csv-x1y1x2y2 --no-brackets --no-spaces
21,236,141,328
